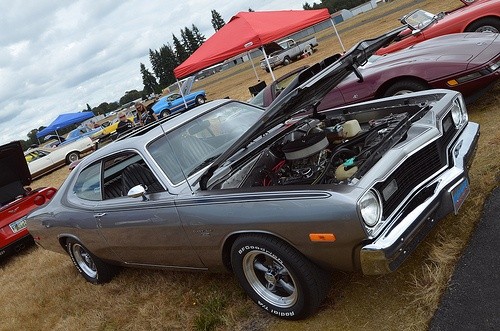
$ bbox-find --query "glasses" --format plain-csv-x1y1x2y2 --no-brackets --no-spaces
137,105,141,109
119,114,125,118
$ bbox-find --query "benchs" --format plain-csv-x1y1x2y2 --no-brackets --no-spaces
102,150,187,197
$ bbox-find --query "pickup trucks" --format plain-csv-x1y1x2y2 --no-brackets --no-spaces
257,36,319,73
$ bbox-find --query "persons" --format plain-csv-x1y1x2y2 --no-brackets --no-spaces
135,102,159,127
117,111,135,137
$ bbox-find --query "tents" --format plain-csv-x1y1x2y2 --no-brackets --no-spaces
36,111,94,145
173,8,346,109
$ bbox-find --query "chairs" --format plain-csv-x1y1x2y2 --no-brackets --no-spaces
249,81,266,97
121,162,163,192
182,135,215,167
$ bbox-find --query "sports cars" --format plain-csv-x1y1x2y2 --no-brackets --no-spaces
25,20,481,323
372,0,500,56
215,31,500,134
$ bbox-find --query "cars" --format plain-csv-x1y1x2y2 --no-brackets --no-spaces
0,76,208,255
